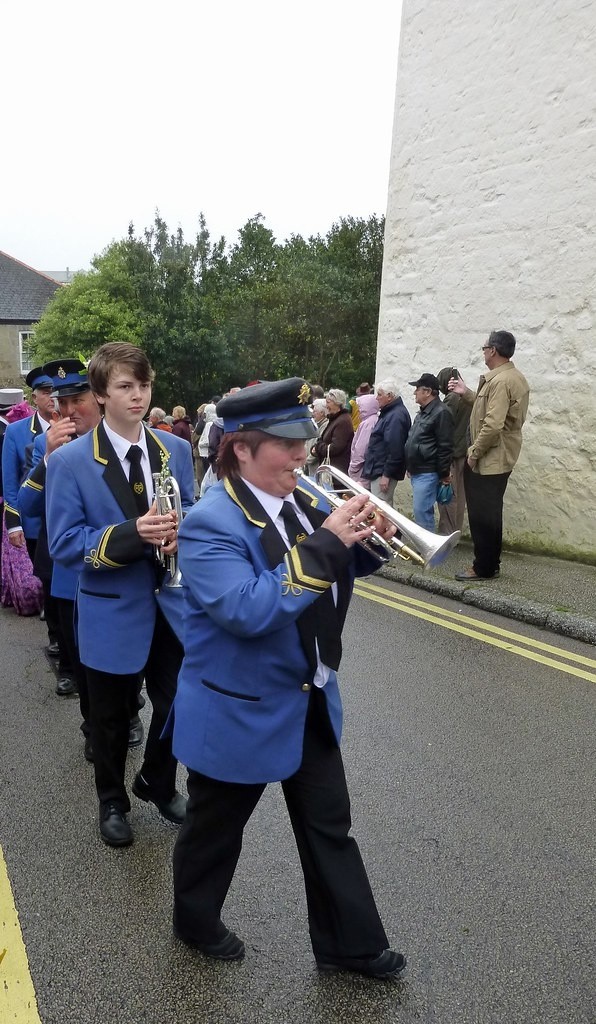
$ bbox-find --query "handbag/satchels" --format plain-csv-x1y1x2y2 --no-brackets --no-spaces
437,482,452,505
319,444,333,492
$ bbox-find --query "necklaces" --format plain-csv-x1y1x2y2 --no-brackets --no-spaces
316,418,326,424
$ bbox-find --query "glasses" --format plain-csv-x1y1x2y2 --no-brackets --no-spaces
329,393,336,399
482,346,491,351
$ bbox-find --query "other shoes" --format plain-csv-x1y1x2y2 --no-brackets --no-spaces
55,676,76,697
187,929,246,961
455,568,500,580
129,715,143,747
139,695,145,703
316,947,408,980
45,642,61,654
86,741,96,760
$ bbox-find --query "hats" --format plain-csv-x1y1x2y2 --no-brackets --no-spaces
216,377,318,439
409,373,440,389
356,382,373,395
313,385,324,399
42,360,88,397
27,365,54,391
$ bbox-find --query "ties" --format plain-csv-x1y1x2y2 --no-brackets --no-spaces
123,444,149,517
280,501,343,673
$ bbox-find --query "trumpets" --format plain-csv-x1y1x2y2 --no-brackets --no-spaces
291,464,463,578
150,472,184,589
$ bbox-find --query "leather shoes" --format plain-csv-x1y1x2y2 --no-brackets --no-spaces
99,802,134,847
133,770,188,824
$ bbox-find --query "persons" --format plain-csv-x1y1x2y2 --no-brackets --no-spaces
158,377,407,978
448,330,530,581
149,366,473,565
2,358,146,762
45,341,194,848
